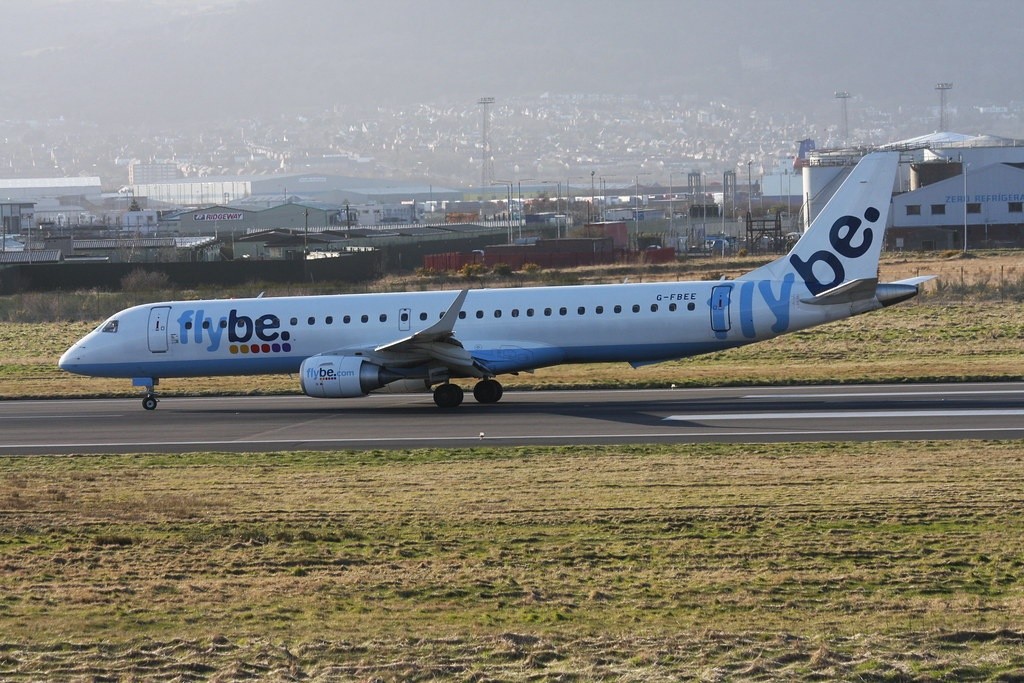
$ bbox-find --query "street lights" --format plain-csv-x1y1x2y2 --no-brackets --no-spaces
590,170,598,222
746,159,752,219
631,175,639,235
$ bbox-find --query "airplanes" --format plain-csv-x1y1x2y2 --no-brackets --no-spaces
58,150,942,414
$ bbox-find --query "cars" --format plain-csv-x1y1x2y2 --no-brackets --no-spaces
688,245,700,253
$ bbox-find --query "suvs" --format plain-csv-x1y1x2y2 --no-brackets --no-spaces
700,240,725,253
